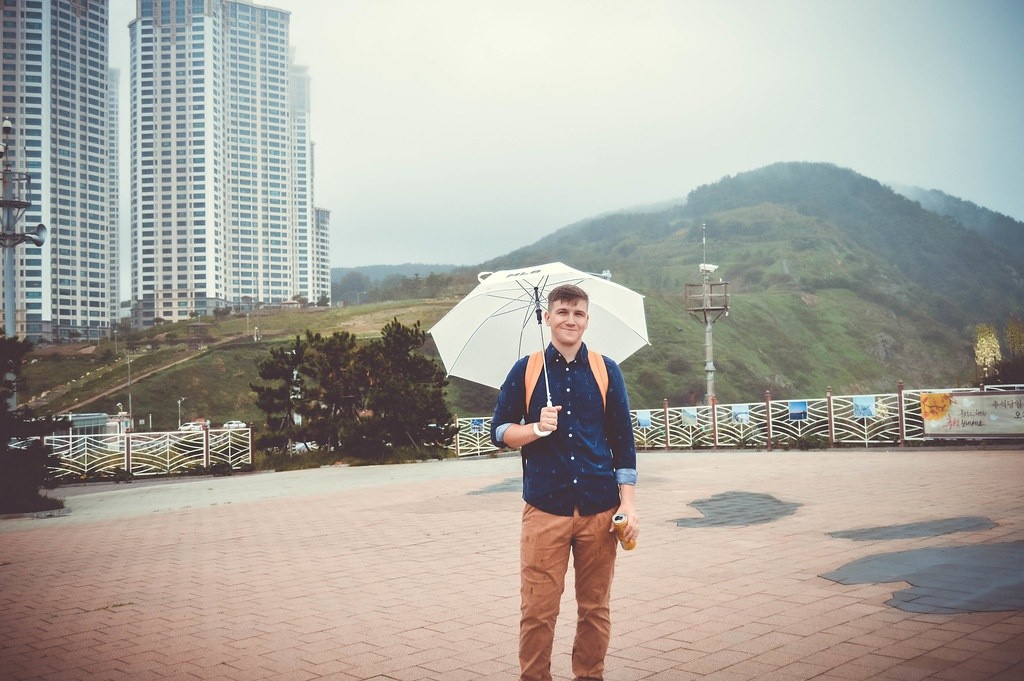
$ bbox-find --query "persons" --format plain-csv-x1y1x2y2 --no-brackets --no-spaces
490,287,641,681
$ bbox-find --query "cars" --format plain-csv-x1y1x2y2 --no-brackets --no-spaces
223,421,246,429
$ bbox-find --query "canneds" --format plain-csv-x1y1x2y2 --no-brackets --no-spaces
612,513,636,550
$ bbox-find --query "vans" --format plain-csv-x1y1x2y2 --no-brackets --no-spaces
178,421,211,431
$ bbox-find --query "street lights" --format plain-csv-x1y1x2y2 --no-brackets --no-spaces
113,330,118,353
117,402,125,433
247,313,250,337
177,397,186,430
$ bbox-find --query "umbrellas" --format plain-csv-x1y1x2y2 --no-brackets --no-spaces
425,259,652,439
